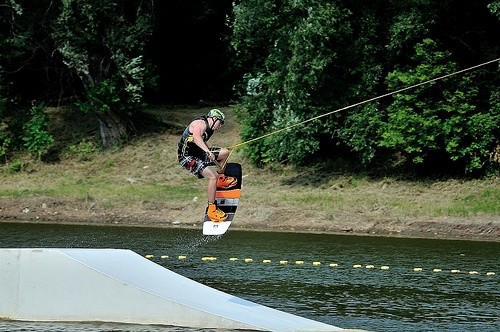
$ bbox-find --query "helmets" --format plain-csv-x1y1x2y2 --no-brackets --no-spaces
207,108,226,123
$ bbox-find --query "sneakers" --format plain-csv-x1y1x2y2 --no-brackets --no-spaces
217,174,237,188
207,208,228,221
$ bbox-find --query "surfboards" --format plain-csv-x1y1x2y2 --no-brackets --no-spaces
202,163,242,235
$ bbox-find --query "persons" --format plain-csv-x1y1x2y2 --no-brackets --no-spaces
178,109,238,222
